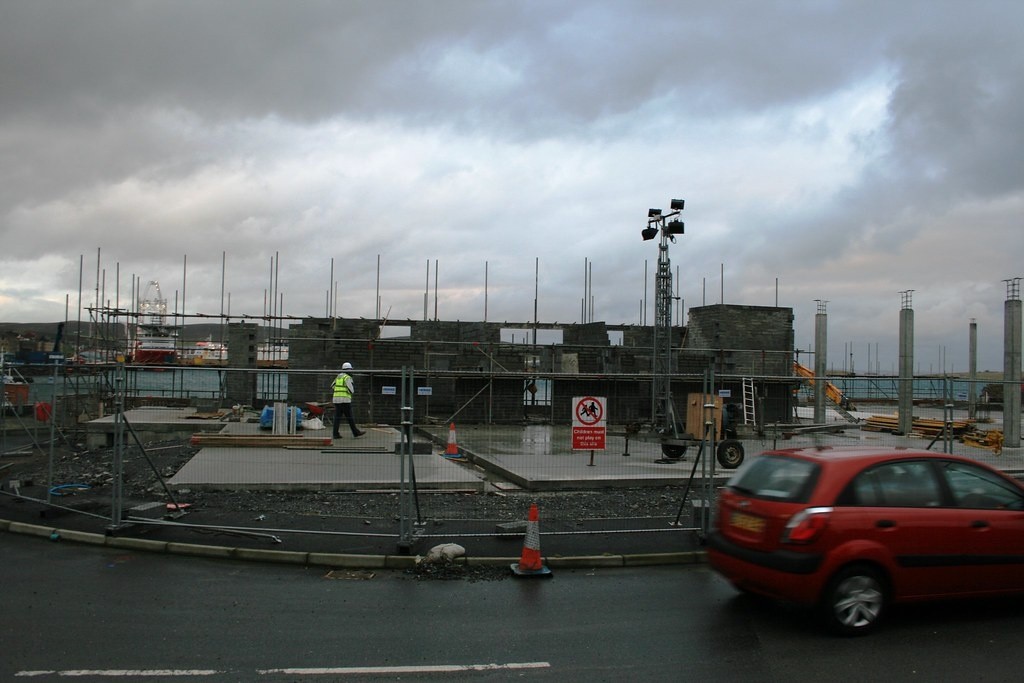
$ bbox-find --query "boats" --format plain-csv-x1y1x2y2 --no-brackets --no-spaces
1,280,290,374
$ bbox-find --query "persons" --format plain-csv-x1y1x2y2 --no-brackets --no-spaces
329,362,367,439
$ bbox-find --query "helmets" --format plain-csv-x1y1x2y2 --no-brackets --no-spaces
342,361,353,370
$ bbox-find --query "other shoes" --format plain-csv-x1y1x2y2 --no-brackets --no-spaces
334,436,343,438
354,431,366,437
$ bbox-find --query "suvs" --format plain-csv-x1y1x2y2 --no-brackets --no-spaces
701,444,1023,634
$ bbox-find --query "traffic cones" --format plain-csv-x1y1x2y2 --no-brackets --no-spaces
509,502,554,580
444,423,459,455
